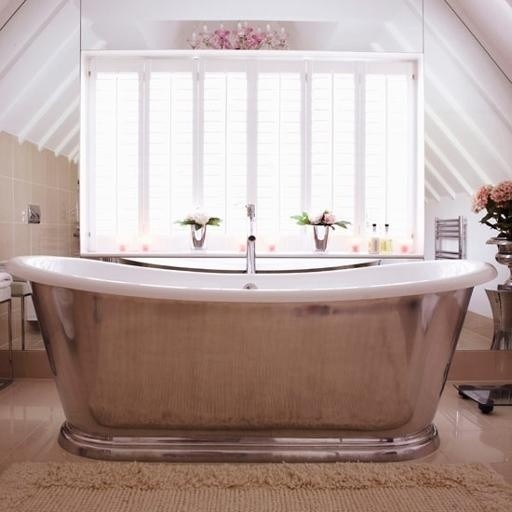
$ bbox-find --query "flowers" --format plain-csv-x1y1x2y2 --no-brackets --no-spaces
292,208,352,232
175,211,222,230
472,179,512,237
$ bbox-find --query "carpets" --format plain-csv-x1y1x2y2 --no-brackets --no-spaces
0,461,512,512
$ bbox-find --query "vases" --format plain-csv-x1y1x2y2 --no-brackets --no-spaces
192,223,208,248
313,226,330,251
487,237,512,290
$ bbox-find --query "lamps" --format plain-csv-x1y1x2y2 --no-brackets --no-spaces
186,22,288,52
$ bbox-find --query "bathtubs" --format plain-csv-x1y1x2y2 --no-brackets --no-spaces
4,254,497,463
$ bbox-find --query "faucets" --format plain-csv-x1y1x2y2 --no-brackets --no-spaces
244,204,256,273
